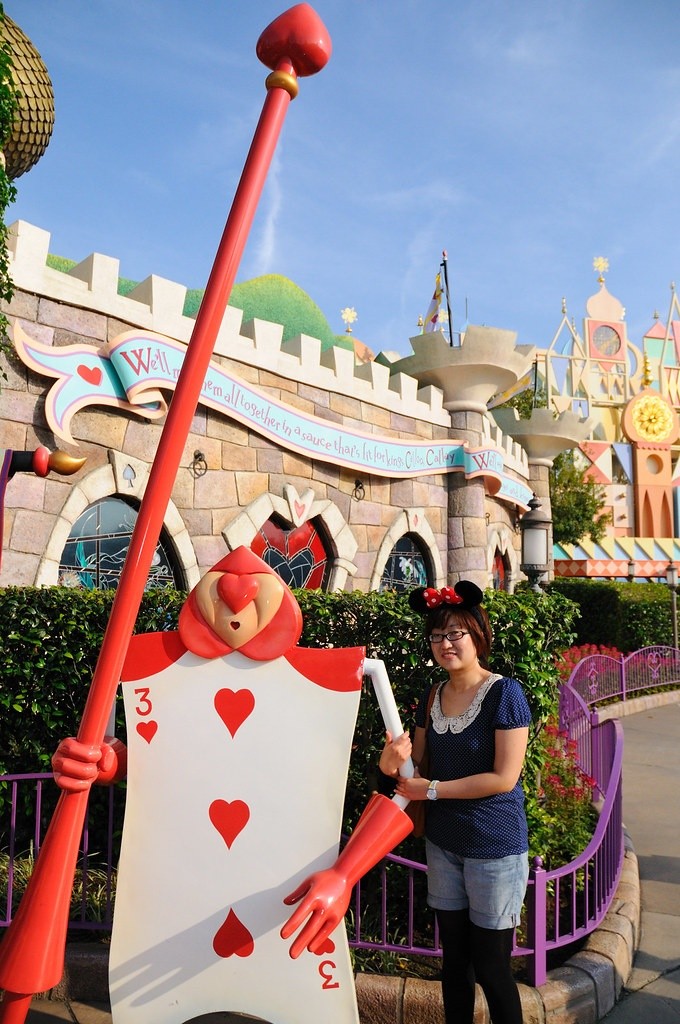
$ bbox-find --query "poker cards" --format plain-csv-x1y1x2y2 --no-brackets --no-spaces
107,629,365,1024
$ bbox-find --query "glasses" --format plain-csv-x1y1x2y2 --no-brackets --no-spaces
429,630,470,643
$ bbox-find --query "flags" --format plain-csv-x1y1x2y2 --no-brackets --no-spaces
421,268,441,334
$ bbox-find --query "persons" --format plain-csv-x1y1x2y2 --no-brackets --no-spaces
379,580,532,1024
51,547,414,1024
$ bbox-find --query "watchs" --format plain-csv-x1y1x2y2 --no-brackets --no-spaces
427,779,440,801
